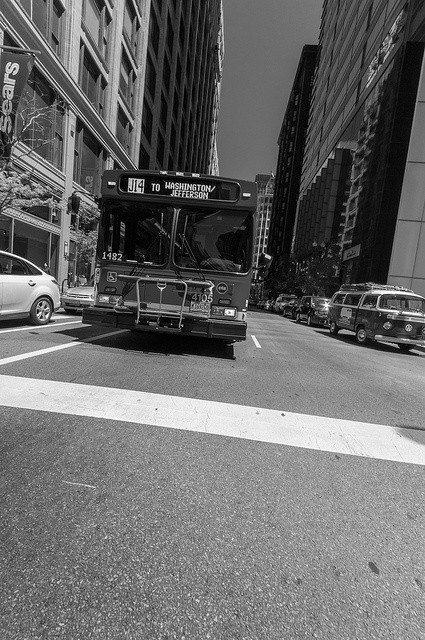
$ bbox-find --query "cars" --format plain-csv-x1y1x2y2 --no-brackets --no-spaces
283,298,299,320
248,297,275,311
1,250,62,327
60,273,97,314
295,294,332,328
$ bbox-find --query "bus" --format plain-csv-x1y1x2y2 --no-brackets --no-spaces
67,167,259,342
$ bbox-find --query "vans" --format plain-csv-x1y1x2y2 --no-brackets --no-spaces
327,281,425,353
275,294,298,315
